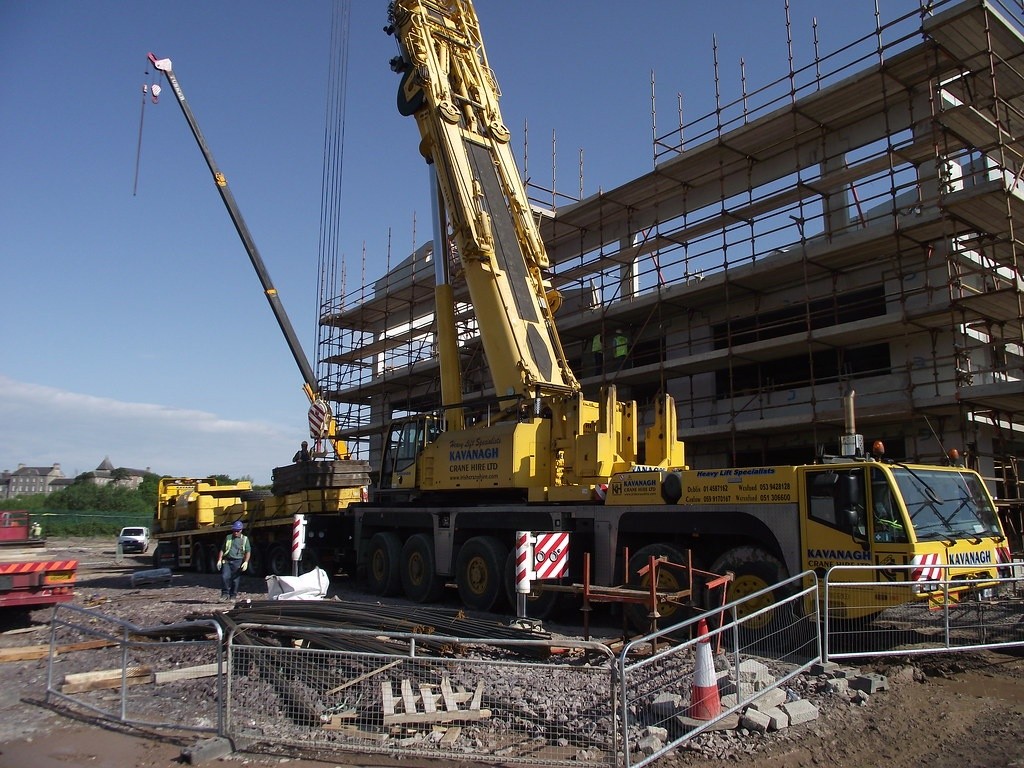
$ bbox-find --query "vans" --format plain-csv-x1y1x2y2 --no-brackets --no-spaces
118,526,151,554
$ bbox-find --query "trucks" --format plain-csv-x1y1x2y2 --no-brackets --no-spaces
0,510,79,633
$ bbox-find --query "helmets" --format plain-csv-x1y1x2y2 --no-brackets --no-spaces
232,521,243,530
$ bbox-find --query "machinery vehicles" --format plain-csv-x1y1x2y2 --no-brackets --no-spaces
353,0,1022,660
131,51,380,580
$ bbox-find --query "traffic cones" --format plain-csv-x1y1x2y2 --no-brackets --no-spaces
676,614,740,734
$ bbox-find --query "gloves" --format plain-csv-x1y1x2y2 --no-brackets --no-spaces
241,561,248,572
216,560,222,571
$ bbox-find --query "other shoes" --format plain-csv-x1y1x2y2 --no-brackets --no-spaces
229,595,236,602
218,595,228,603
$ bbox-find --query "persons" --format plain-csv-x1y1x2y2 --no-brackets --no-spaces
217,521,251,602
32,522,42,538
293,441,310,464
612,329,628,357
592,334,606,375
